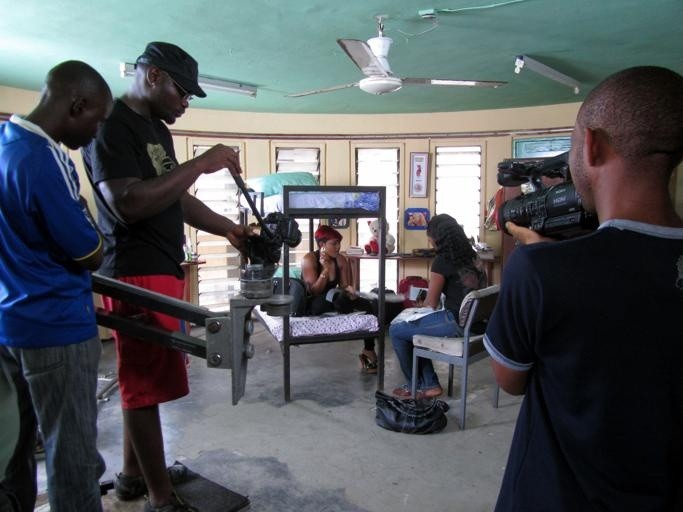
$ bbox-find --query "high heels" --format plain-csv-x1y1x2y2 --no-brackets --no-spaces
359,353,383,375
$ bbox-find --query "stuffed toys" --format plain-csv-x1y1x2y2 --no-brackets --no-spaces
365,220,395,256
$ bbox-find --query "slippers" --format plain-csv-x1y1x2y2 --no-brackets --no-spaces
394,384,427,400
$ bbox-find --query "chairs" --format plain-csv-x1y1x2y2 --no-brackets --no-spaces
410,283,501,431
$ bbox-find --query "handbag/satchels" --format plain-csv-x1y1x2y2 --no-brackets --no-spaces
272,277,307,317
374,391,449,435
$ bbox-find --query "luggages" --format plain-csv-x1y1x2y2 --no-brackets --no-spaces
398,276,428,309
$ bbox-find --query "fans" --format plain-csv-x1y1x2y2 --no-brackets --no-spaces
281,16,509,101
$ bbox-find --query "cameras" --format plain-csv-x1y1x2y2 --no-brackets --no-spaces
249,211,302,248
497,150,599,241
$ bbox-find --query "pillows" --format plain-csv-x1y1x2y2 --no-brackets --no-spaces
274,268,303,279
260,172,319,197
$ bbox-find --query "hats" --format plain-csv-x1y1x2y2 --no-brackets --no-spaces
133,42,206,99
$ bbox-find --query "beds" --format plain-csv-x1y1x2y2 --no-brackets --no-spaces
233,171,386,402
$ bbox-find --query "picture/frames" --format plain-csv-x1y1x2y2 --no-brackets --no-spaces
409,152,429,197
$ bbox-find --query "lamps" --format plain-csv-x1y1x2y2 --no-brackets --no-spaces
514,55,582,94
123,63,257,100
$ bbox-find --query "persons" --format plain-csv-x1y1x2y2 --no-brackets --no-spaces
1,60,112,511
301,225,378,367
483,65,682,512
388,214,489,401
82,42,258,510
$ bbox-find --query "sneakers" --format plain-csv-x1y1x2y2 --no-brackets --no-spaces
142,491,197,512
115,465,187,502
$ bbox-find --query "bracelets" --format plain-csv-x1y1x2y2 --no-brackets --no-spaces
319,273,327,279
